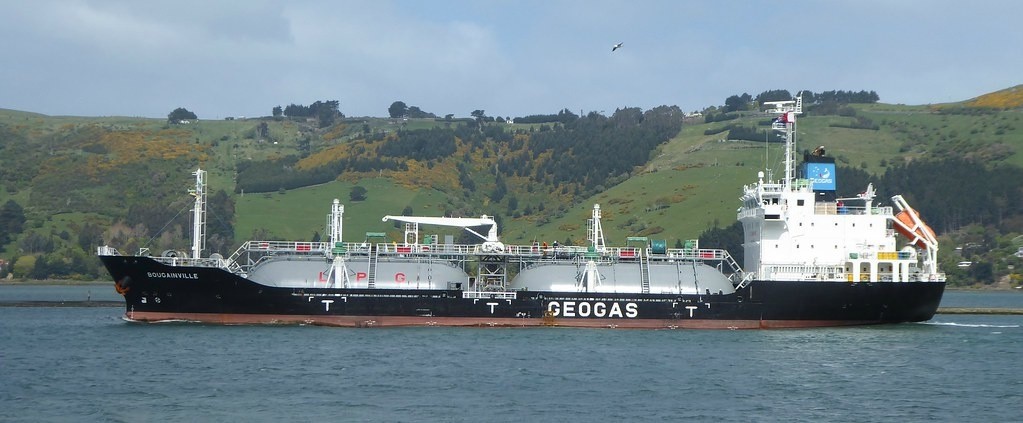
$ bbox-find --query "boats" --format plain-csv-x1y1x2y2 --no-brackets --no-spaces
892,209,937,249
96,89,948,330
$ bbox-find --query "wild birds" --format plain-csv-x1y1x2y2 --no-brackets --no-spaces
611,42,623,52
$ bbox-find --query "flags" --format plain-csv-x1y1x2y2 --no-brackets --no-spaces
771,111,794,123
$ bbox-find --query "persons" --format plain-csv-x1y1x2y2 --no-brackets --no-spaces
553,241,559,259
543,242,548,256
